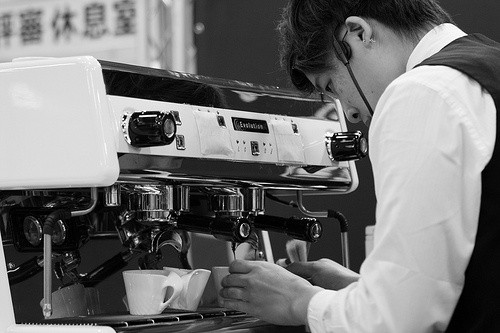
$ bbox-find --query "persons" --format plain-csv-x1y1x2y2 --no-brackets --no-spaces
220,0,500,333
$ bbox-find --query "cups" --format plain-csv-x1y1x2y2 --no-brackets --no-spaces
123,269,183,316
162,266,212,312
213,267,230,307
41,284,102,320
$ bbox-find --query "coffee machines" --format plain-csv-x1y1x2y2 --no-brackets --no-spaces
0,57,368,333
